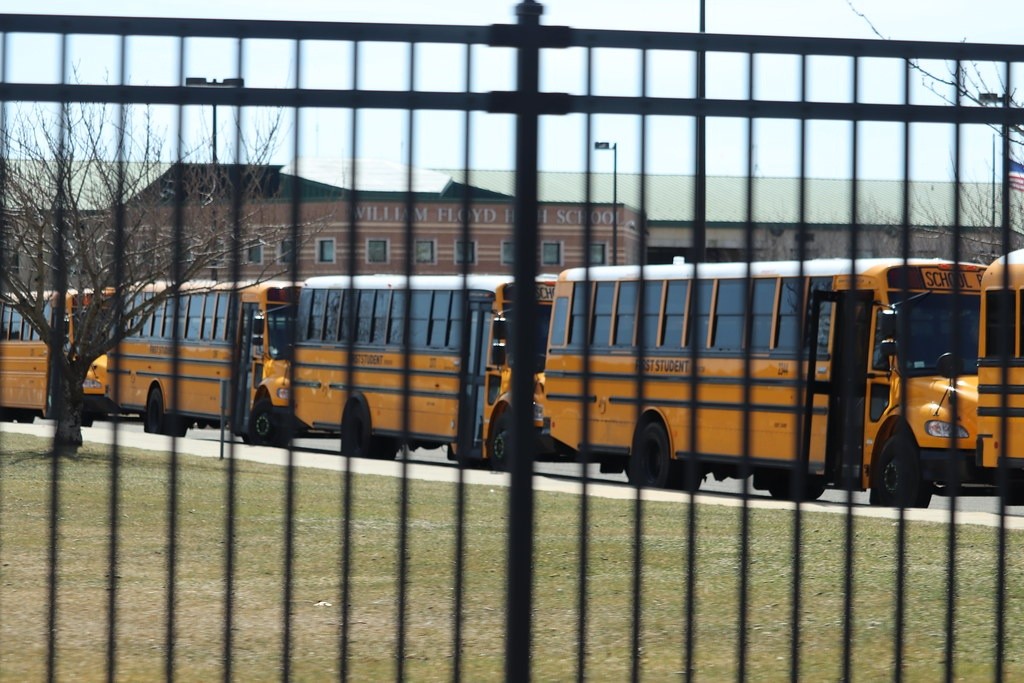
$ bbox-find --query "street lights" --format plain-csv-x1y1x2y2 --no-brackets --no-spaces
594,139,619,264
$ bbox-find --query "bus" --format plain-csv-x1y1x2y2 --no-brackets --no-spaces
101,278,301,444
540,254,991,508
0,287,108,425
973,246,1024,506
291,271,558,471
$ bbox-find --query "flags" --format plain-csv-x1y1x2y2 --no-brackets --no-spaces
1008,159,1024,192
